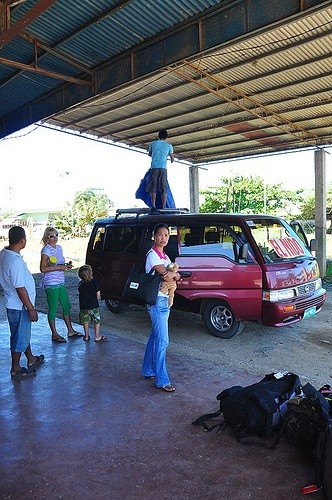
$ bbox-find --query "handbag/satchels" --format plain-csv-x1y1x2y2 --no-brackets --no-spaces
121,266,159,307
191,368,304,450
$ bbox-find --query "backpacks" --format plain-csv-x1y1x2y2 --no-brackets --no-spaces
284,381,332,500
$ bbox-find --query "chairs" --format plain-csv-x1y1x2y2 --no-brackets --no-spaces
169,235,178,242
205,232,220,244
185,233,199,245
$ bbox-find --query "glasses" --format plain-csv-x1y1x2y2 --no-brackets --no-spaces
48,233,59,239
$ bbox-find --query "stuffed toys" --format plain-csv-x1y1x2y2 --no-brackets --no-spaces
159,262,179,306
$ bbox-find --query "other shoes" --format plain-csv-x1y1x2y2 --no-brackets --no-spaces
160,384,176,393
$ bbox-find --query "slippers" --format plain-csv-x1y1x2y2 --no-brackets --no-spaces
82,335,91,341
68,332,84,339
51,336,68,343
27,354,45,369
10,367,37,378
95,335,107,342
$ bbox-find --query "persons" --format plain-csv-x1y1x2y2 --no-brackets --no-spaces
77,265,107,342
0,226,44,378
148,129,174,211
140,223,181,392
39,227,84,342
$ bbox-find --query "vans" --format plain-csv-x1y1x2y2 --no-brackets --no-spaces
86,208,332,339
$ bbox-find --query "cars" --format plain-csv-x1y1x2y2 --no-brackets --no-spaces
0,235,9,242
32,227,38,232
57,229,92,241
